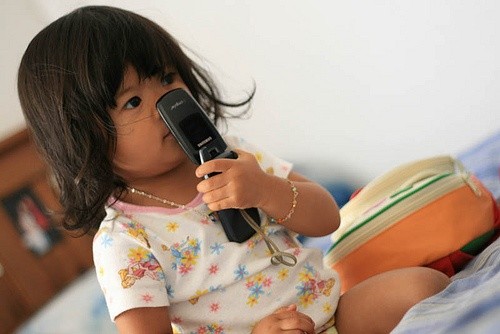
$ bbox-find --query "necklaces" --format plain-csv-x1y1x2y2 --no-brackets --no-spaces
118,184,214,220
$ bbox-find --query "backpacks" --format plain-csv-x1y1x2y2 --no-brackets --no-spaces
323,155,496,295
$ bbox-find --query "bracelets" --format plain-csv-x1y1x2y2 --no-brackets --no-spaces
267,179,298,224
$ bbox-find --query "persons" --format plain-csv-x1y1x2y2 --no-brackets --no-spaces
19,5,450,333
